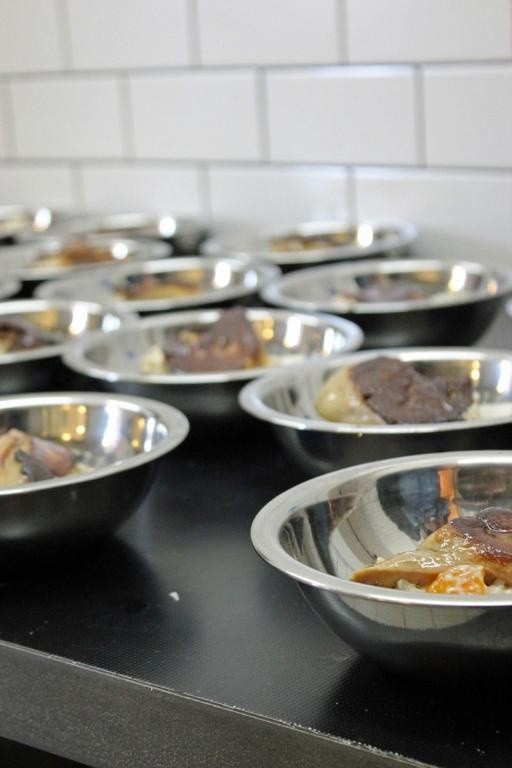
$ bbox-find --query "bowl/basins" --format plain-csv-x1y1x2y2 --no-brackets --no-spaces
0,297,121,371
65,307,366,420
266,257,512,342
249,449,512,675
0,389,192,566
1,198,418,314
233,344,512,487
